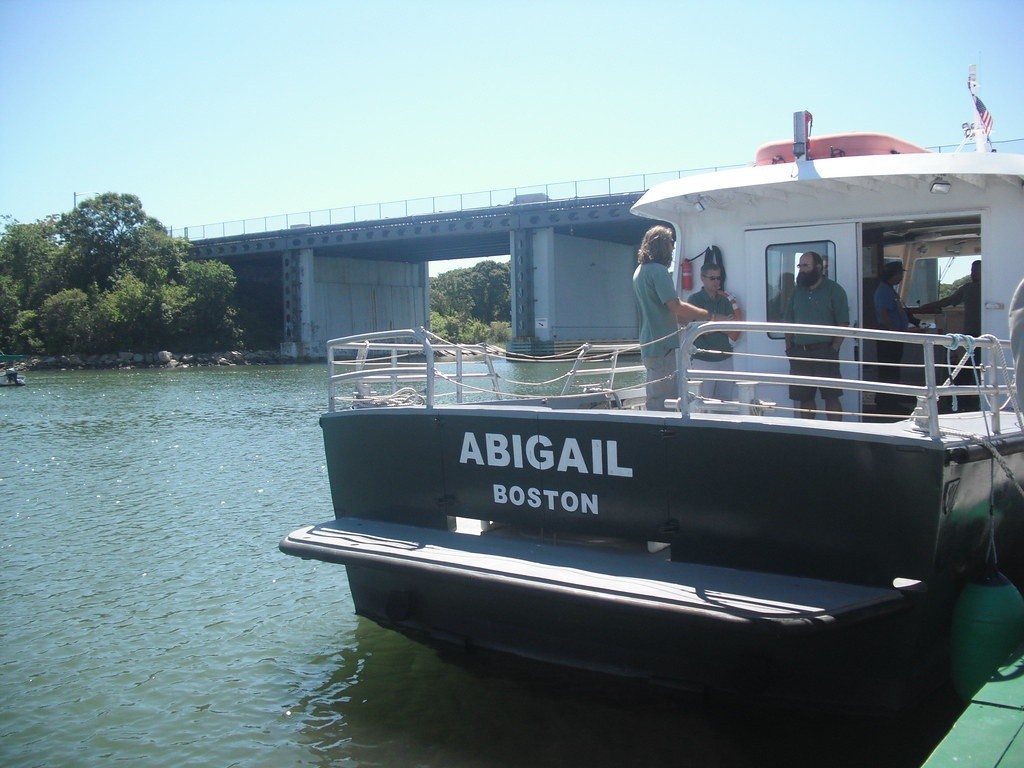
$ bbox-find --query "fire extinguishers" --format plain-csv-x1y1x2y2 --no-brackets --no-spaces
682,258,692,289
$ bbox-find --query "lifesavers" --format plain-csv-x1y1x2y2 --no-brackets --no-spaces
716,288,742,346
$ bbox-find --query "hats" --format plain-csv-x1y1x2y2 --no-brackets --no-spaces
884,261,907,271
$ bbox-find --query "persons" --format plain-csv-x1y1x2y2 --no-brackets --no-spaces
905,260,980,412
677,263,742,415
870,258,931,415
785,252,849,421
632,226,737,412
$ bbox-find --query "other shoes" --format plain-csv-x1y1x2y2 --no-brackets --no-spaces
877,404,911,414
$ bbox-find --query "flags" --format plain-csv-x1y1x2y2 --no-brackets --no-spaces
968,81,994,140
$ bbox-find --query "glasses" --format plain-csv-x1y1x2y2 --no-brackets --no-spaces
703,275,722,281
797,264,816,268
669,239,675,246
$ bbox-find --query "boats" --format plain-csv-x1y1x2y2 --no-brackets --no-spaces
277,110,1024,730
0,367,27,386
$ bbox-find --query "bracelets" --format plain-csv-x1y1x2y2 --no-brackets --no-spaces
832,347,837,352
785,348,790,352
707,310,715,321
